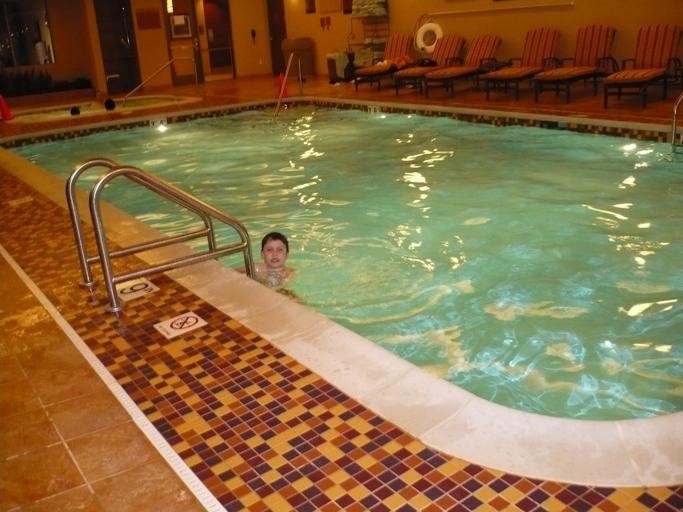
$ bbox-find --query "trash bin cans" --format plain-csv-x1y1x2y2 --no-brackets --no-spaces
34,40,48,65
281,38,316,78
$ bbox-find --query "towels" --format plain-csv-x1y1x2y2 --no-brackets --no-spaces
325,51,349,78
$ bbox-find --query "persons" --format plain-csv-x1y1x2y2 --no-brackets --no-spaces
236,233,298,293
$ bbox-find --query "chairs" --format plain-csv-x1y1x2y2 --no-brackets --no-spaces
479,26,560,100
392,32,468,100
423,32,502,100
602,22,683,108
533,22,621,104
353,29,418,93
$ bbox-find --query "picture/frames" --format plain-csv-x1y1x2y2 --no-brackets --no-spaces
167,13,194,40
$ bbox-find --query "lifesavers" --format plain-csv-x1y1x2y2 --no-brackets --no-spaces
416,22,443,53
